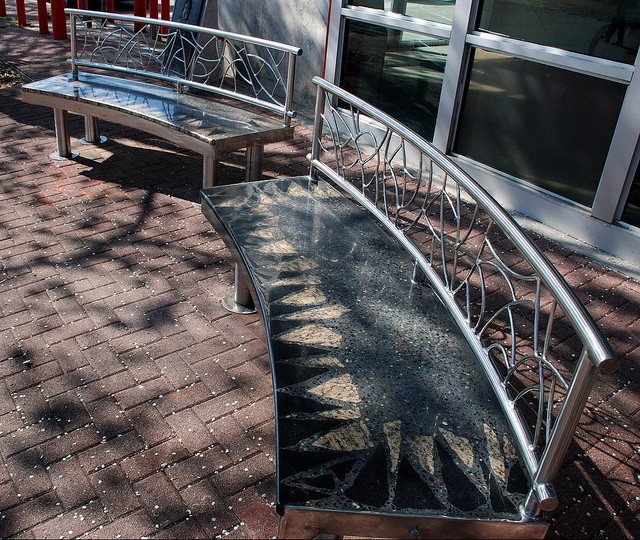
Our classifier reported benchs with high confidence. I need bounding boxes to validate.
[200,74,619,540]
[22,8,302,188]
